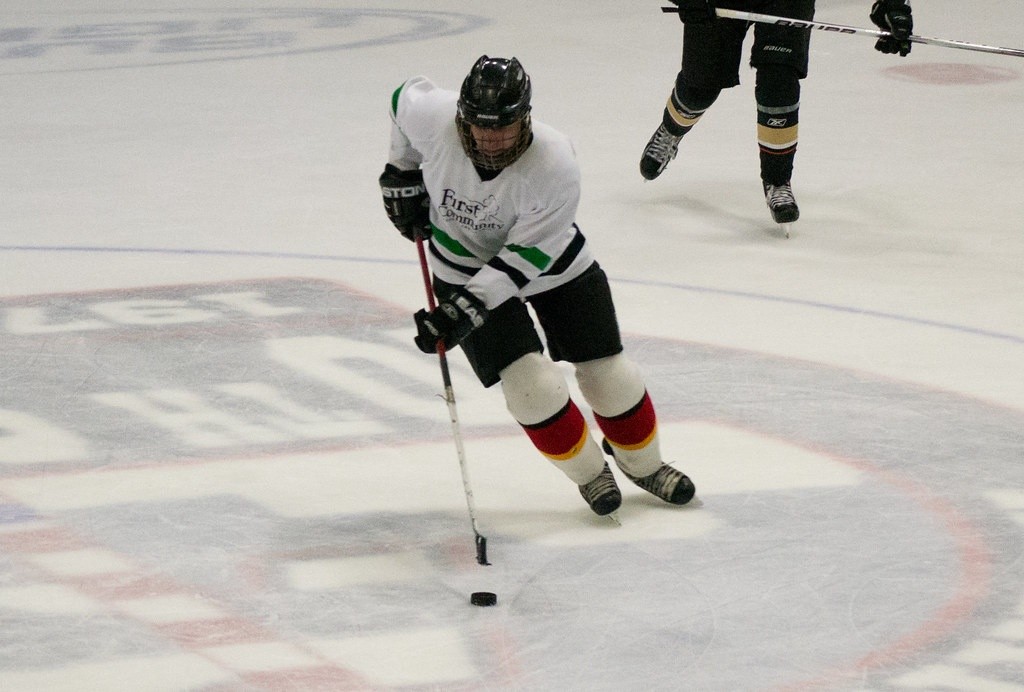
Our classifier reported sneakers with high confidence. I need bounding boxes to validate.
[639,121,683,183]
[602,438,696,506]
[578,461,622,527]
[763,180,800,238]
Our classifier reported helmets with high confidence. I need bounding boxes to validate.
[457,55,532,130]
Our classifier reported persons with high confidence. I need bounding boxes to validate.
[640,0,913,224]
[377,53,697,516]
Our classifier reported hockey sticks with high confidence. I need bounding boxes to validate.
[714,7,1024,60]
[411,227,500,565]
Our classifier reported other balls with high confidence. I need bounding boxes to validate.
[471,592,497,606]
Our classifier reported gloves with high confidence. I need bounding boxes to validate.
[668,0,717,26]
[379,172,433,242]
[870,0,913,57]
[413,287,489,354]
